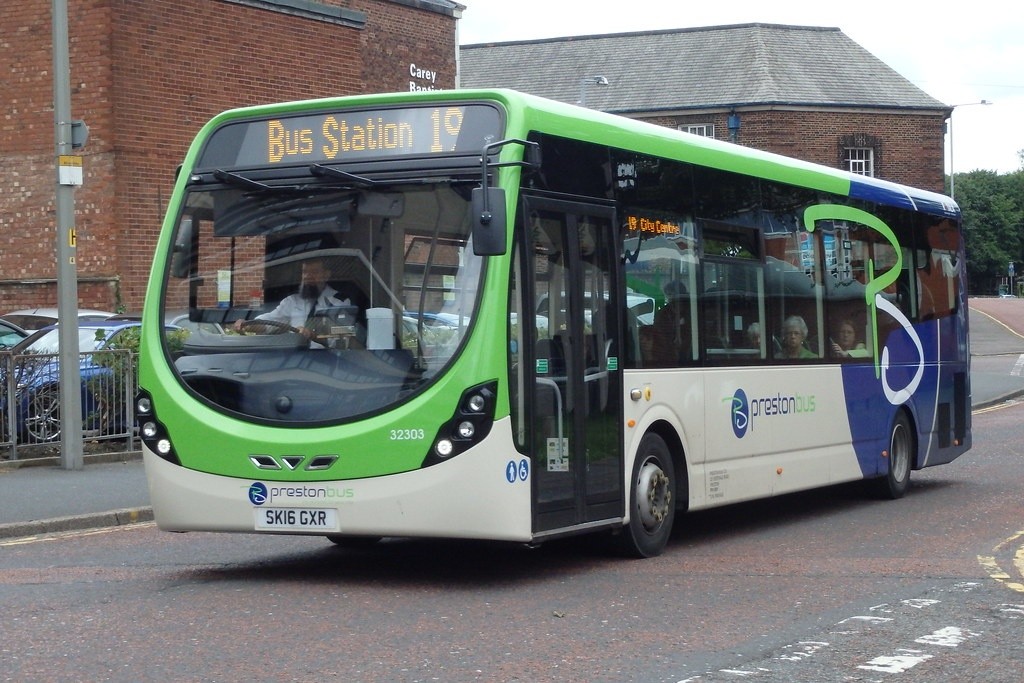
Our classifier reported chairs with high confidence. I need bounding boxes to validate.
[333,278,372,319]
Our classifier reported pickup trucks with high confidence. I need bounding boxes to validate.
[401,316,458,360]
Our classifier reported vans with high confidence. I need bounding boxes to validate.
[537,289,655,327]
[133,84,970,562]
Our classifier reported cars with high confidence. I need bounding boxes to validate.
[401,311,472,326]
[0,321,183,444]
[104,311,225,339]
[0,308,122,347]
[0,318,41,368]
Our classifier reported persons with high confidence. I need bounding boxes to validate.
[638,266,901,364]
[229,246,351,350]
